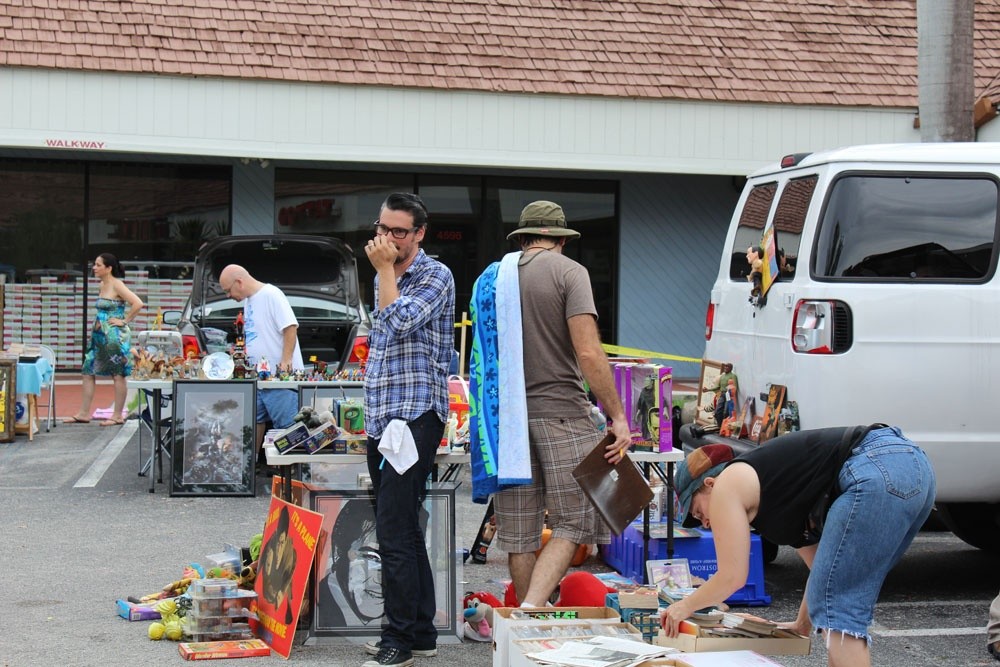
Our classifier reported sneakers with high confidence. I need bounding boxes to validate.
[364,642,416,666]
[365,636,436,656]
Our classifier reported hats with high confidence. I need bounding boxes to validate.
[671,446,734,528]
[507,201,581,243]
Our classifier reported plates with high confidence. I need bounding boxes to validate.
[204,351,235,380]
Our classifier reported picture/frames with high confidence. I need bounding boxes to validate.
[0,358,15,443]
[301,480,464,646]
[694,357,727,427]
[167,378,257,498]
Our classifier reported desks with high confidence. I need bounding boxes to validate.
[125,373,364,494]
[262,427,686,592]
[13,358,48,439]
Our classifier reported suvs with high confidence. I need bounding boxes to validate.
[161,233,372,381]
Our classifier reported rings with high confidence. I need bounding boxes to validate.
[368,244,374,247]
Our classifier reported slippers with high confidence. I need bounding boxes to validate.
[99,419,122,427]
[64,415,89,423]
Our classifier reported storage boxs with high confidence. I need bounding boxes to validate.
[176,541,272,661]
[303,361,339,372]
[598,352,672,454]
[490,512,813,667]
[200,327,231,353]
[258,394,369,455]
[0,268,194,370]
[115,598,161,621]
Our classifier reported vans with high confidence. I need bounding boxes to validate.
[698,140,1000,549]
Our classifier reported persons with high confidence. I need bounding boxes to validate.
[63,253,143,426]
[320,499,429,628]
[218,264,305,477]
[658,424,936,667]
[469,201,632,607]
[265,505,291,610]
[364,194,454,664]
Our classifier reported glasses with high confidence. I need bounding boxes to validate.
[223,281,237,294]
[374,221,419,239]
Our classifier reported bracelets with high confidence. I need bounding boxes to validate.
[122,319,127,325]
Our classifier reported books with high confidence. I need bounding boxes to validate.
[662,609,776,638]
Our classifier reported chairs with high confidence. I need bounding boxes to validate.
[133,388,171,477]
[25,343,57,432]
